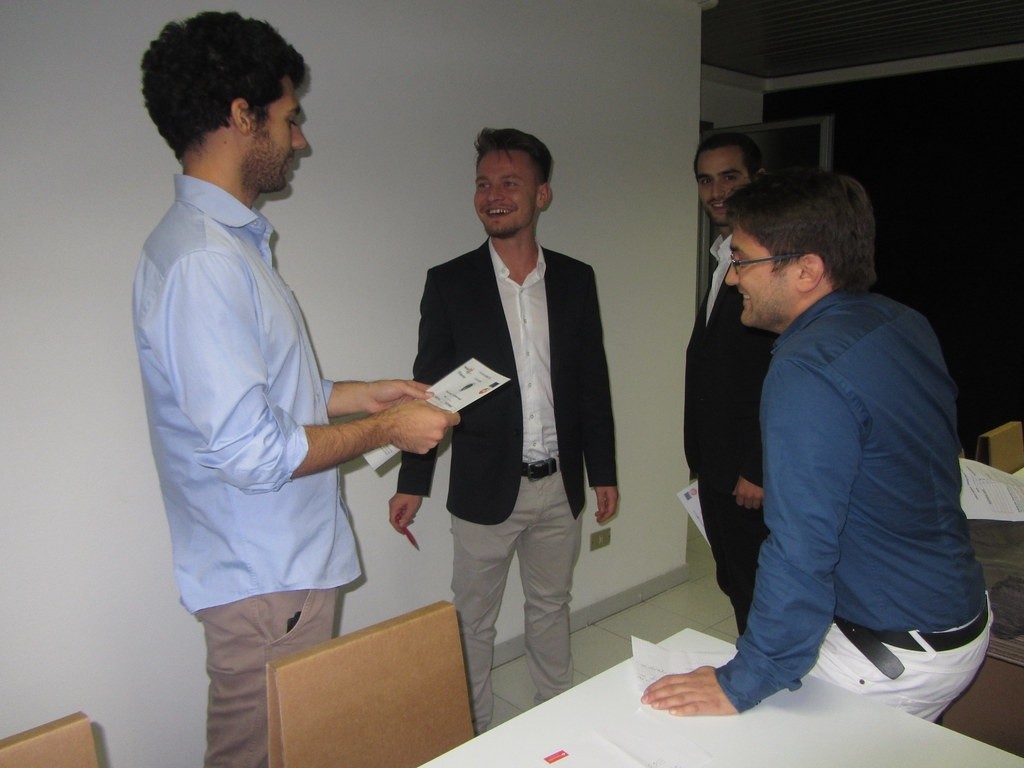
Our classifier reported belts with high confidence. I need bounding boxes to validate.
[835,592,997,686]
[520,456,559,481]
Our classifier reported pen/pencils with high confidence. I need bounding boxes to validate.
[395,515,422,552]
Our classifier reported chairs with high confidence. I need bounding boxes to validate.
[272,602,474,768]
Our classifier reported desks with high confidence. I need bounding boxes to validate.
[416,628,1024,768]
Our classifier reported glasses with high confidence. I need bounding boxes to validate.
[729,250,806,275]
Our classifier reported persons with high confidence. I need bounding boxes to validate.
[682,128,783,648]
[390,126,622,743]
[129,11,460,768]
[639,168,996,728]
